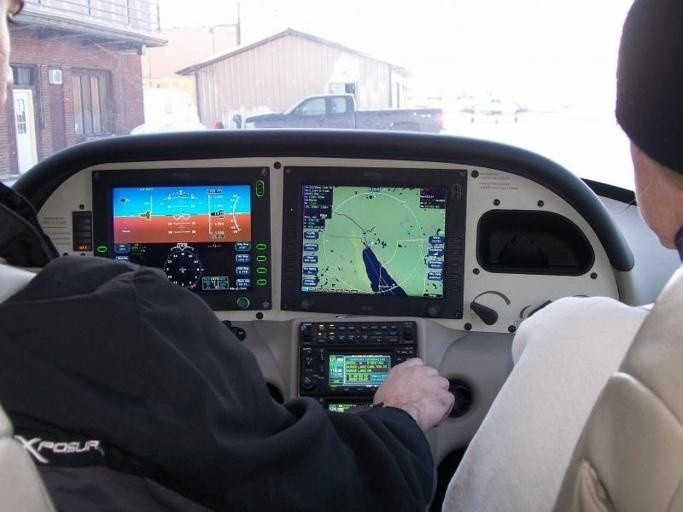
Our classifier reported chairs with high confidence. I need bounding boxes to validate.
[551,263,683,512]
[0,404,58,511]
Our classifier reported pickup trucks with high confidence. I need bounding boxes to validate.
[241,92,446,135]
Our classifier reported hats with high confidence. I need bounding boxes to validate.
[616,0,683,175]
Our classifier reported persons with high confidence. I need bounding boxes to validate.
[1,0,460,512]
[436,0,680,511]
